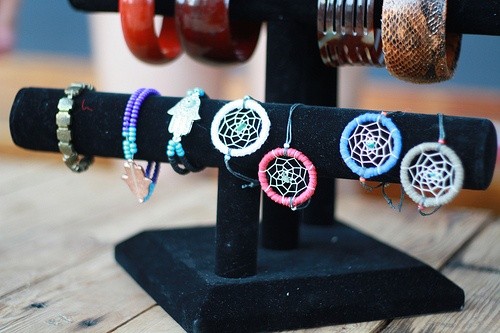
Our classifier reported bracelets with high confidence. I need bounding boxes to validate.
[175,0,264,67]
[401,112,466,217]
[167,84,211,175]
[339,110,402,212]
[382,0,464,84]
[317,0,386,69]
[260,103,318,211]
[123,87,167,206]
[120,0,186,67]
[56,83,96,173]
[212,95,272,188]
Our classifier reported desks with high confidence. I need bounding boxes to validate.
[0,154,500,333]
